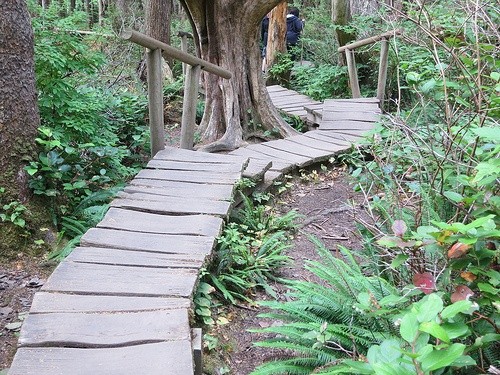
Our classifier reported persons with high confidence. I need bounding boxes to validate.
[261,12,268,72]
[286,7,305,68]
